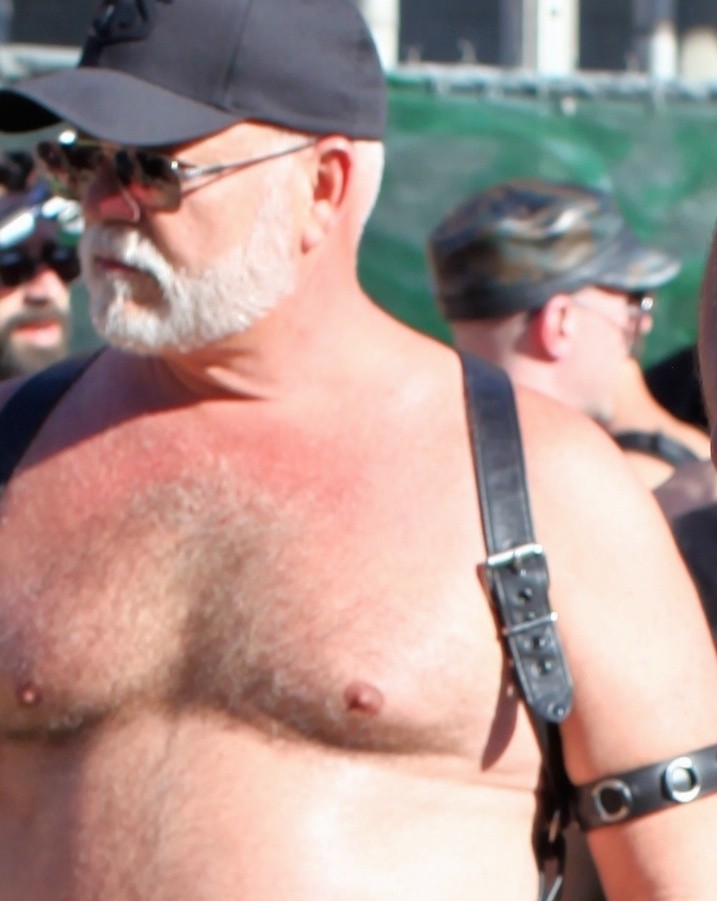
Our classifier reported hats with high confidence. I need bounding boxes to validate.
[0,0,388,148]
[426,181,683,322]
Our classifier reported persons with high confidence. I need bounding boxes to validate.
[0,0,717,901]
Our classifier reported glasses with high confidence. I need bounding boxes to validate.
[37,138,328,213]
[568,292,654,321]
[0,242,80,286]
